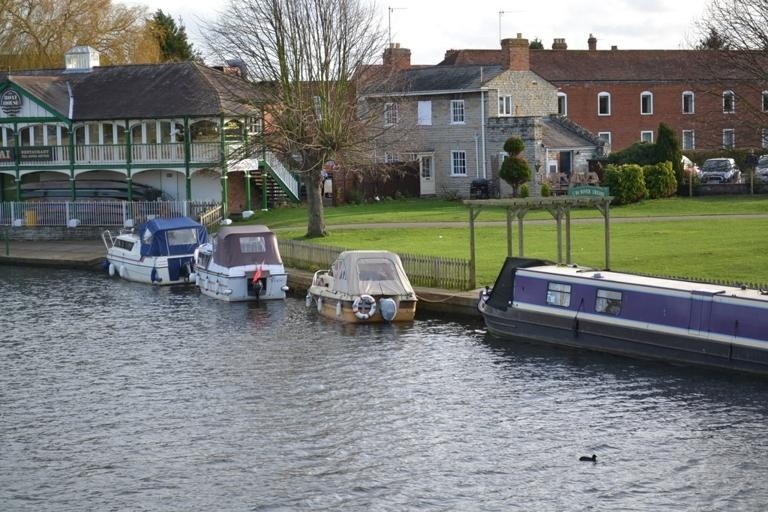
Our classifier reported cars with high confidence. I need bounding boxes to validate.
[697,157,740,184]
[680,155,699,180]
[752,155,768,192]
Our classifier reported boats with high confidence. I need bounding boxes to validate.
[104,215,209,286]
[478,256,767,381]
[193,222,289,303]
[307,249,417,324]
